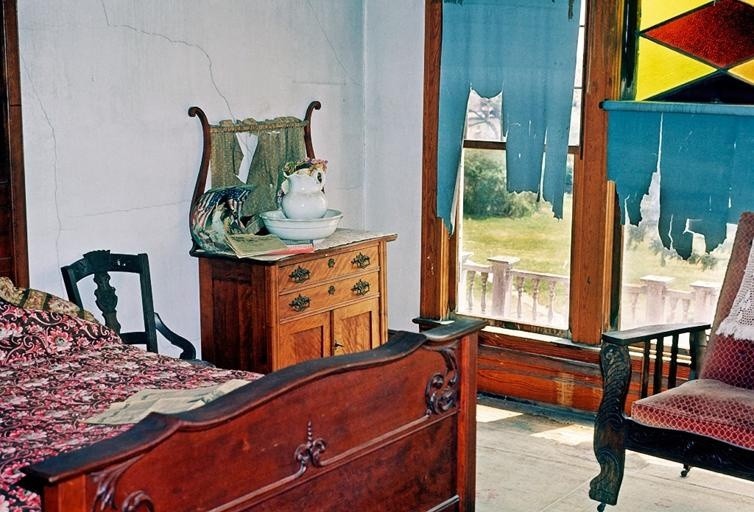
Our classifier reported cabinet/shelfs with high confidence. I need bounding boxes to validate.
[198,233,403,376]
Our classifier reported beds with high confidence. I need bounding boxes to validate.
[0,271,492,512]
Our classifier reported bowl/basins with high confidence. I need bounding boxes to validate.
[258,208,344,241]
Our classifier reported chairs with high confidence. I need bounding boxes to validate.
[59,249,197,365]
[586,210,752,511]
